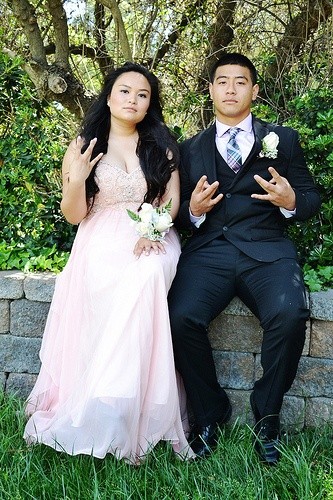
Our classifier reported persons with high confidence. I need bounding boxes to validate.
[166,53,322,465]
[23,60,197,466]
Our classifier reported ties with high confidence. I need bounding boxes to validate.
[225,126,245,174]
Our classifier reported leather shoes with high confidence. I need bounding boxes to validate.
[250,393,283,467]
[187,406,232,461]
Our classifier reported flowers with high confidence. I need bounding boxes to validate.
[259,132,279,160]
